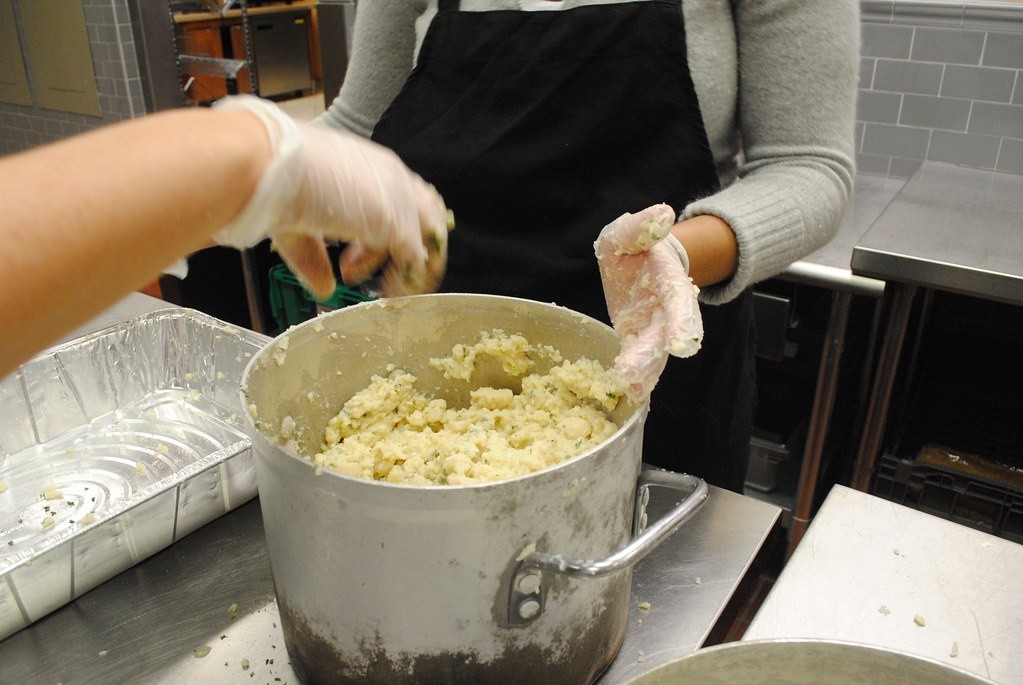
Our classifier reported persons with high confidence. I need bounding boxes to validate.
[297,3,863,499]
[1,93,454,384]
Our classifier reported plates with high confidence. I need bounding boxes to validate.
[1,307,279,645]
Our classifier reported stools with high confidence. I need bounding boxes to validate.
[728,485,1022,685]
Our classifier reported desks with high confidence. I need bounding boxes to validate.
[770,168,906,572]
[2,290,787,685]
[849,155,1022,543]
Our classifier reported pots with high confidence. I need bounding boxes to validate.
[238,293,711,685]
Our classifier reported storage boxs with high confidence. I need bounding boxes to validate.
[0,305,279,643]
